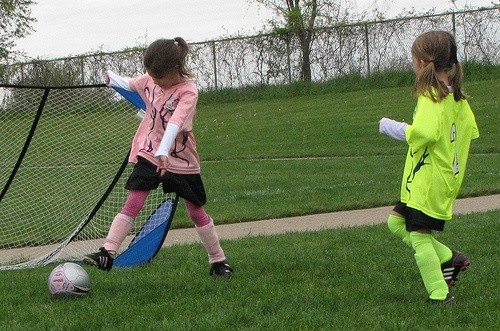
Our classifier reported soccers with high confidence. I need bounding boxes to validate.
[48,262,91,300]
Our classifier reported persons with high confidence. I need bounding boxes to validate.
[378,31,480,305]
[82,36,236,281]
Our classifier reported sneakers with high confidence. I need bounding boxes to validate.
[425,292,457,306]
[441,249,470,290]
[212,258,232,282]
[83,246,114,272]
